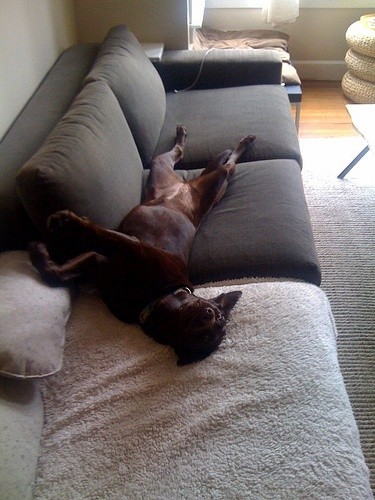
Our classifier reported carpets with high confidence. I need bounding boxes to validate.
[297,137,375,497]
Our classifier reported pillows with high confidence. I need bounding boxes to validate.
[14,79,146,266]
[82,24,166,170]
[0,249,73,381]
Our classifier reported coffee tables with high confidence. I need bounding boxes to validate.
[338,104,375,179]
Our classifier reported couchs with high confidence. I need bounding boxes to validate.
[0,40,375,500]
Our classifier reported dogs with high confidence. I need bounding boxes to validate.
[27,124,255,366]
